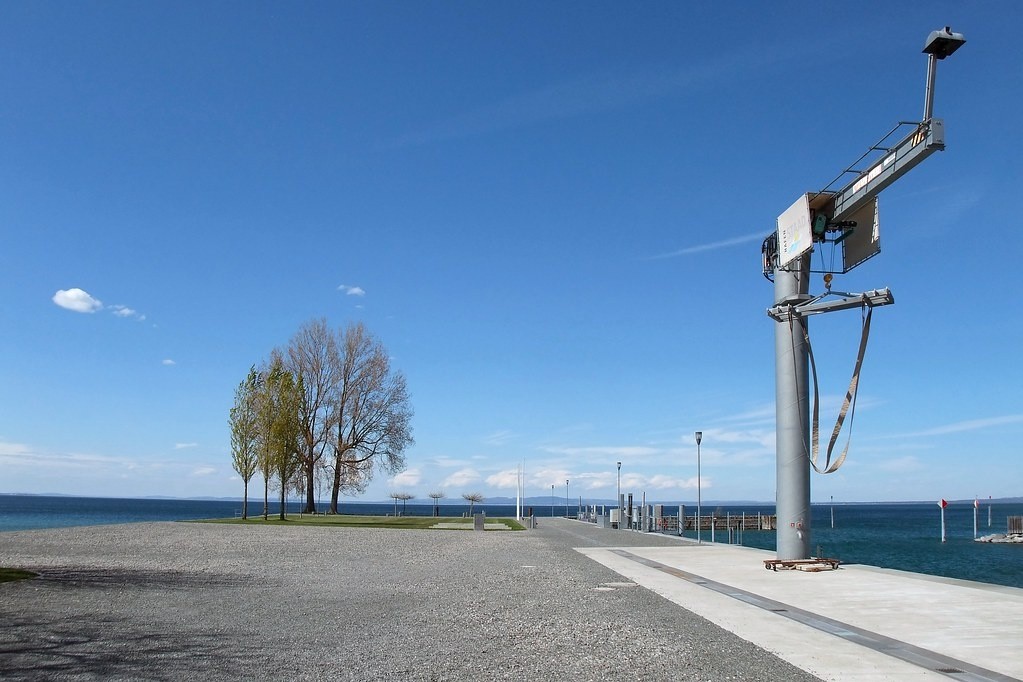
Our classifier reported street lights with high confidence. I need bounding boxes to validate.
[695,432,702,545]
[761,24,967,564]
[551,485,554,517]
[617,461,621,530]
[566,479,569,521]
